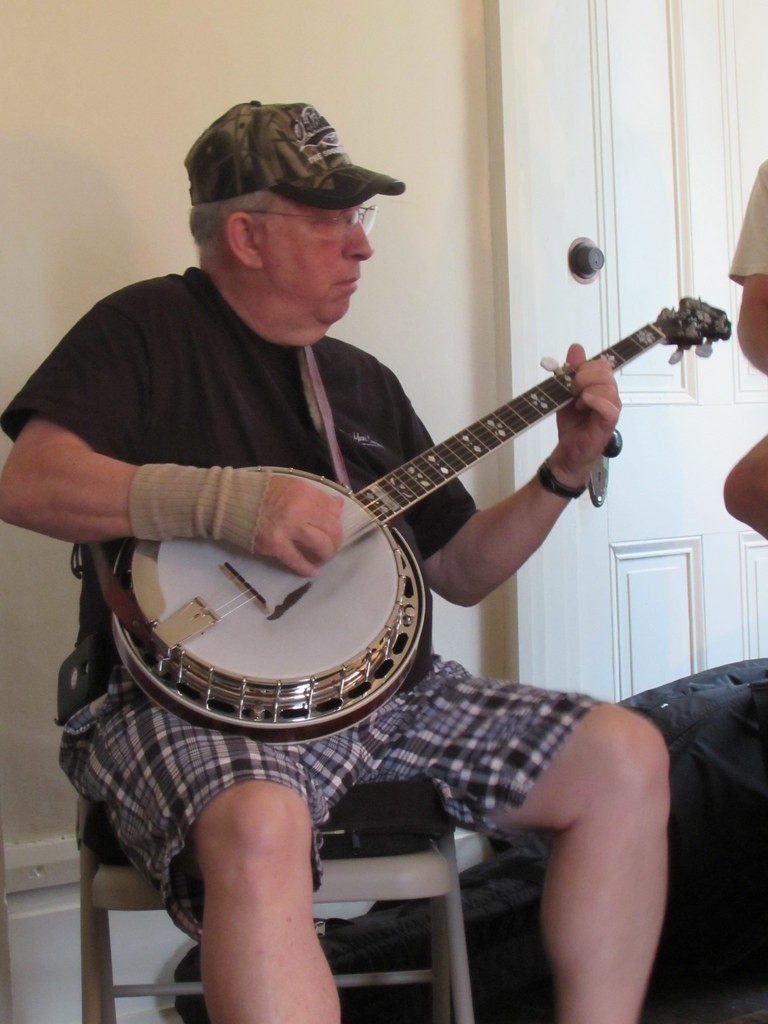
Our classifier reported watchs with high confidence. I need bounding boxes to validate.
[538,460,590,500]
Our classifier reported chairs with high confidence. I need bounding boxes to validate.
[73,790,476,1024]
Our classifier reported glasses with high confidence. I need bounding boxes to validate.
[245,204,378,236]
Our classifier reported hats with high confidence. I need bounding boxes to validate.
[183,101,406,208]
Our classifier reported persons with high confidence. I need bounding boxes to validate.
[723,156,768,541]
[0,102,670,1024]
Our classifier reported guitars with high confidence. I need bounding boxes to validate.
[110,294,730,742]
